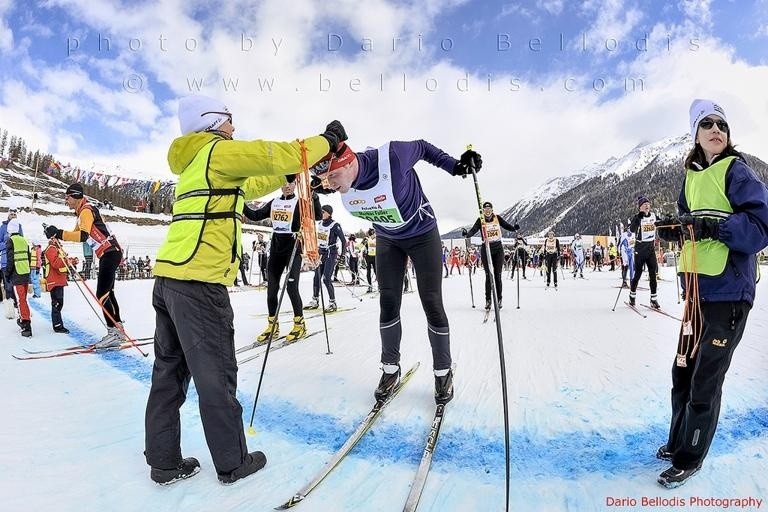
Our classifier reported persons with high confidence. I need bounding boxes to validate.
[629,196,663,309]
[309,139,482,403]
[245,177,323,341]
[305,204,345,312]
[49,184,125,349]
[655,100,767,489]
[144,95,347,485]
[1,237,150,300]
[461,202,520,309]
[1,211,24,304]
[2,219,33,338]
[234,226,634,296]
[42,225,78,334]
[263,185,267,188]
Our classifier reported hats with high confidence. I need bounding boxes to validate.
[321,204,333,215]
[178,91,231,137]
[483,201,493,208]
[636,196,650,206]
[66,181,82,199]
[691,98,731,147]
[6,208,19,234]
[308,138,356,176]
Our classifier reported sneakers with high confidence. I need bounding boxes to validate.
[304,298,338,313]
[375,362,400,402]
[152,457,200,486]
[347,278,372,292]
[17,319,69,337]
[547,282,558,287]
[32,295,41,299]
[258,317,279,342]
[650,293,660,309]
[215,451,268,486]
[434,369,454,405]
[286,316,306,341]
[485,299,503,310]
[656,441,702,488]
[629,293,637,305]
[93,322,124,350]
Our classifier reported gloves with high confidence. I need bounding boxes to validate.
[455,148,482,176]
[320,119,348,153]
[653,215,721,243]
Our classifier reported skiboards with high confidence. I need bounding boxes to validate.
[625,301,682,324]
[483,308,489,323]
[235,329,327,364]
[251,304,357,323]
[13,336,155,360]
[276,363,457,511]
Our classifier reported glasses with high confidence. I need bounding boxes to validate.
[64,193,79,199]
[305,147,348,174]
[200,111,233,123]
[698,116,729,133]
